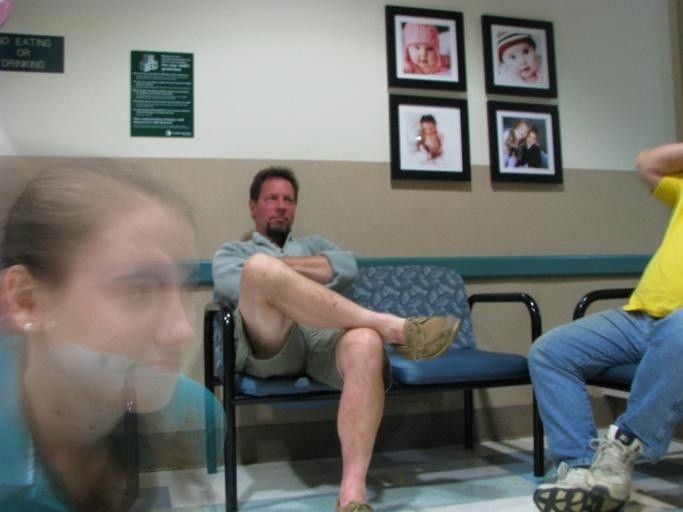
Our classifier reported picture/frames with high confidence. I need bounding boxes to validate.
[486,99,564,184]
[481,12,558,98]
[384,3,467,91]
[389,93,471,182]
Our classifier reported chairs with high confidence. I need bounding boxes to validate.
[203,265,548,512]
[569,287,683,394]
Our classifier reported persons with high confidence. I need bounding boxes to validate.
[416,113,449,163]
[0,158,227,512]
[496,28,546,84]
[212,166,460,512]
[528,140,683,511]
[402,23,450,75]
[503,120,550,168]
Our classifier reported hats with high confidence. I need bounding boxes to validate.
[403,23,442,72]
[496,31,536,64]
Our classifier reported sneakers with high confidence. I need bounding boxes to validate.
[334,496,374,512]
[585,423,646,502]
[391,315,461,361]
[532,461,631,511]
